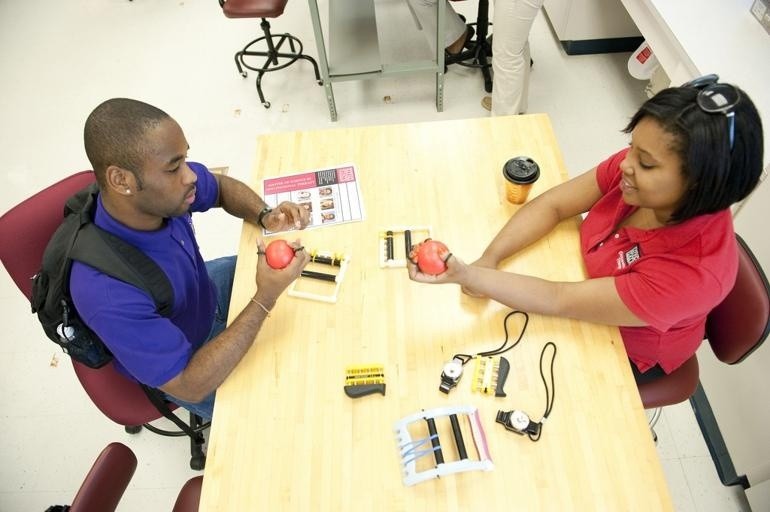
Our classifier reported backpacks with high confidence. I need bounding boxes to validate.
[30,185,175,369]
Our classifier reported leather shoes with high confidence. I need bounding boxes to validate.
[482,96,492,107]
[444,25,474,63]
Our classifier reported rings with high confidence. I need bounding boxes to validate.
[256,251,266,255]
[293,246,305,252]
[444,252,452,265]
[408,244,419,264]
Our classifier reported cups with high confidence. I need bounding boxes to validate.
[503,155,541,205]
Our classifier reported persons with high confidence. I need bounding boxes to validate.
[403,1,544,118]
[70,96,311,425]
[296,187,337,227]
[405,72,764,383]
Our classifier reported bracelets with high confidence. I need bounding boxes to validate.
[251,298,270,314]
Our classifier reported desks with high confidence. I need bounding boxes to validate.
[306,1,454,126]
[167,111,680,512]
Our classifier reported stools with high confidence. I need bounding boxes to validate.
[442,0,535,97]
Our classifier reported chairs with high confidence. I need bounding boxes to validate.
[626,229,770,449]
[0,168,208,472]
[216,0,325,114]
[64,440,204,512]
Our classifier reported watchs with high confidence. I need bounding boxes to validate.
[258,206,272,229]
[497,407,542,438]
[440,355,465,393]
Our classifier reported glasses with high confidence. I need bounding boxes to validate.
[687,73,740,152]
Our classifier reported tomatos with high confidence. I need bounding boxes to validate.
[265,239,294,270]
[417,240,451,276]
